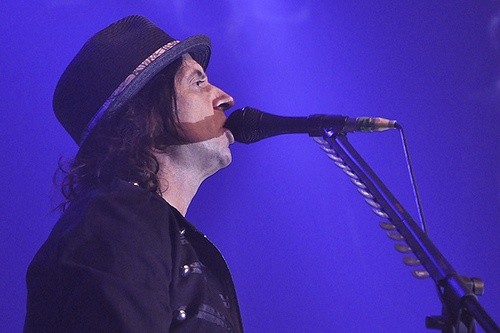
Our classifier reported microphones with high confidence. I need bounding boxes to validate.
[228,106,398,144]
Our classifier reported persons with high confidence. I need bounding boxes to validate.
[23,16,245,333]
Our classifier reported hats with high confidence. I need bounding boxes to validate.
[52,14,212,155]
